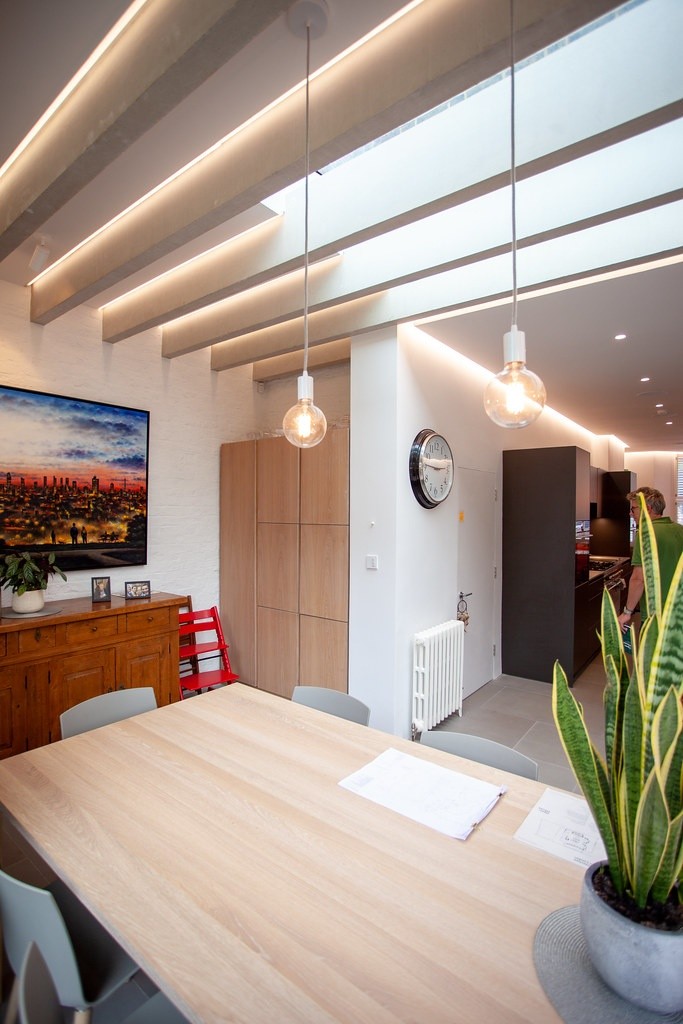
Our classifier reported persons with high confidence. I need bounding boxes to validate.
[95,582,109,601]
[127,584,149,597]
[51,523,116,544]
[617,487,683,634]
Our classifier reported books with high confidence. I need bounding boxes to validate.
[621,624,633,655]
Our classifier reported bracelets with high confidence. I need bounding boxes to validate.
[623,606,633,615]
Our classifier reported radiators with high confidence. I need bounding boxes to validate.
[413,618,462,742]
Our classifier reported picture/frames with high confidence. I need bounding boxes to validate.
[92,577,112,603]
[124,581,151,600]
[0,384,151,570]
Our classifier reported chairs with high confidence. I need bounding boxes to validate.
[291,686,370,727]
[1,872,141,1024]
[177,607,240,700]
[420,731,539,783]
[59,687,160,741]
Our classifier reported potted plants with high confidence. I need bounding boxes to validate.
[1,552,67,613]
[551,490,683,1013]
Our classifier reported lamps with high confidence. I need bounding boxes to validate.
[484,0,549,427]
[282,1,327,448]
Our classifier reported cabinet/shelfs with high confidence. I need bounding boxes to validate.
[0,589,194,758]
[502,446,636,689]
[219,424,349,701]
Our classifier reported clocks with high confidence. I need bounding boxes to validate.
[409,428,454,509]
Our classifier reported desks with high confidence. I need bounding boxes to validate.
[0,682,596,1023]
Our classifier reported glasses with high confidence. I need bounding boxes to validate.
[630,506,639,513]
